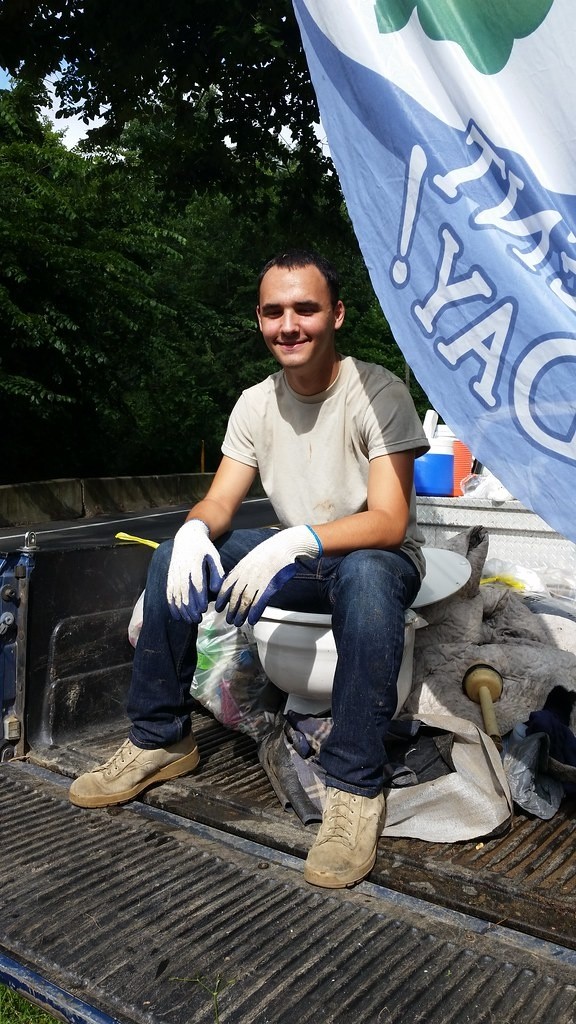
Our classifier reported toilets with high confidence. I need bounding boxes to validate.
[252,606,416,726]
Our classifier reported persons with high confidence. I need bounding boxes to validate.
[69,254,432,888]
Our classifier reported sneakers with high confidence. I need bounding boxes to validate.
[303,786,387,888]
[69,730,199,808]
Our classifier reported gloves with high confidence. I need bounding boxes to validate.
[166,518,226,625]
[214,523,323,627]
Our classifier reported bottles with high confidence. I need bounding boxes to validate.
[191,626,220,696]
